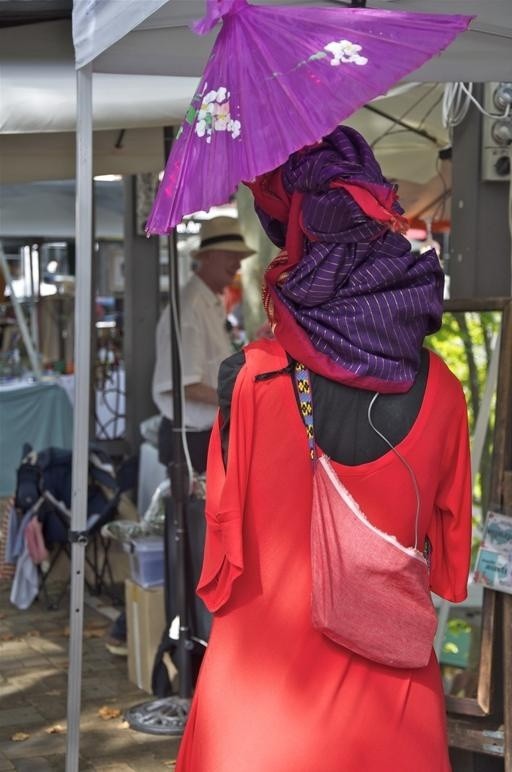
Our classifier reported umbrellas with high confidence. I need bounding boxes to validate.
[143,1,474,241]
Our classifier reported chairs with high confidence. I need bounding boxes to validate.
[8,451,137,612]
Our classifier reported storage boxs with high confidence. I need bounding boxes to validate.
[123,577,168,691]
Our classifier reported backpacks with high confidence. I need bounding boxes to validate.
[15,443,119,549]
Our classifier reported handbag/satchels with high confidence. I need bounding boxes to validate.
[312,456,438,669]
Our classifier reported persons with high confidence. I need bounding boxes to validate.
[173,125,475,771]
[149,214,257,700]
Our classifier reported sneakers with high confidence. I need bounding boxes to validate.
[106,638,128,655]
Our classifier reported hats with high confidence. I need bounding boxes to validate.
[190,217,257,259]
